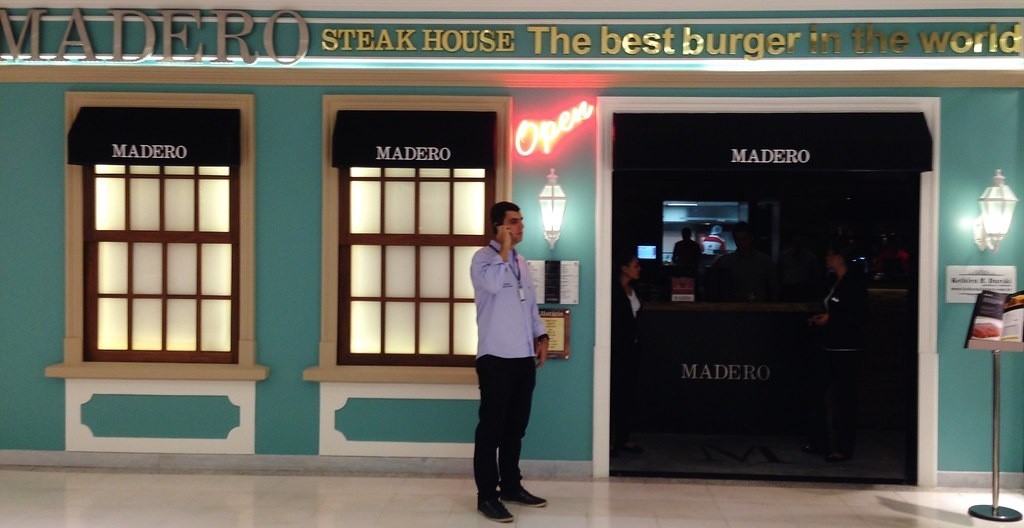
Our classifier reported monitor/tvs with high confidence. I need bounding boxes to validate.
[637,245,657,260]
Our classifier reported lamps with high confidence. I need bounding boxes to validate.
[973,169,1020,255]
[538,168,568,251]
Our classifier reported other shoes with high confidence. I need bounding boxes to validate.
[825,440,853,463]
[801,438,828,453]
[611,443,618,458]
[615,437,637,450]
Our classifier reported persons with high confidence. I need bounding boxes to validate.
[470,201,549,522]
[672,227,705,266]
[802,235,868,463]
[610,250,642,459]
[705,221,774,303]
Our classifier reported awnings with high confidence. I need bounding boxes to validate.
[67,106,241,167]
[613,112,932,173]
[332,110,497,168]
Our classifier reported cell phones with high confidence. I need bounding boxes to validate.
[493,224,501,234]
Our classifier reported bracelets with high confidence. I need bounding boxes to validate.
[537,334,549,344]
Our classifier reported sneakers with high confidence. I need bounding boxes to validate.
[500,487,547,507]
[476,496,514,522]
